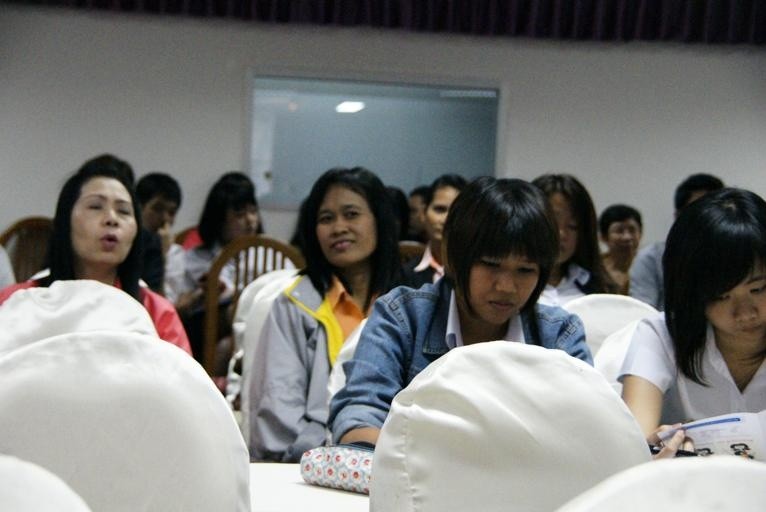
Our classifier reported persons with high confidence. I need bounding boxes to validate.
[249,167,412,464]
[617,186,766,436]
[410,174,723,310]
[0,155,270,375]
[328,176,594,442]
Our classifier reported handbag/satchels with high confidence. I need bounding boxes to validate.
[299,441,375,494]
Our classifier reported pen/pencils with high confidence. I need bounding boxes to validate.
[649,444,698,457]
